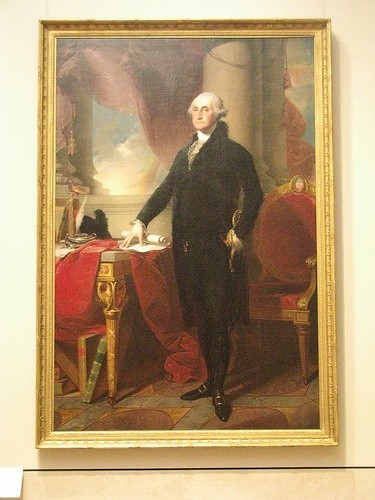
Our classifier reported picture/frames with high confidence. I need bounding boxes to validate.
[35,19,339,449]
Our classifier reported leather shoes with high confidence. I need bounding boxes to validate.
[180,381,209,400]
[209,393,228,424]
